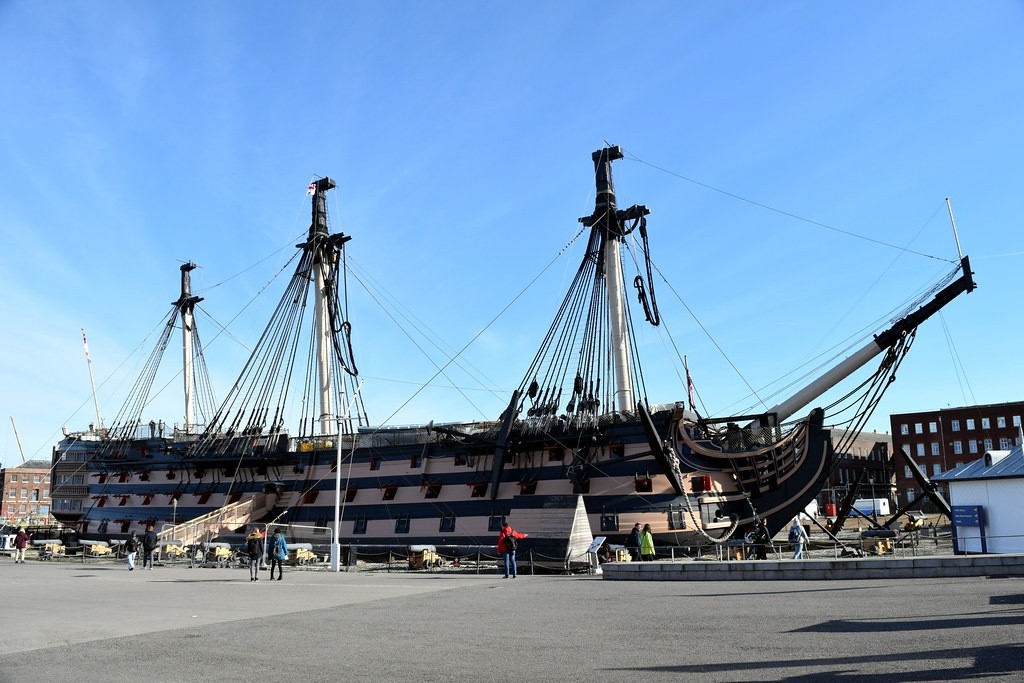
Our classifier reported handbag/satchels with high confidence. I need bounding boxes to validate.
[148,538,157,549]
[268,546,280,560]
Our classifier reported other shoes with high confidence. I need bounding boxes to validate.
[15,561,18,563]
[513,576,516,579]
[270,577,275,580]
[277,577,282,580]
[148,568,153,570]
[130,568,133,571]
[503,576,509,579]
[255,577,258,580]
[21,561,25,563]
[251,578,254,581]
[141,567,145,569]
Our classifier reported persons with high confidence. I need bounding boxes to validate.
[624,522,655,561]
[744,517,772,560]
[268,527,288,580]
[141,525,158,569]
[147,419,165,438]
[13,527,30,563]
[124,530,140,571]
[246,527,264,582]
[790,518,809,559]
[497,522,529,578]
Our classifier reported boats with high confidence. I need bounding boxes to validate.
[861,529,899,538]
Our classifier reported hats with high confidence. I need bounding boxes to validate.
[274,528,281,533]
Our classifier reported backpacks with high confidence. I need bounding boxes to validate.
[624,536,632,550]
[500,530,517,551]
[789,527,801,543]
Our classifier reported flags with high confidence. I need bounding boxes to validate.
[82,331,91,362]
[688,375,696,408]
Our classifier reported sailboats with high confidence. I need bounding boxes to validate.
[47,145,978,575]
